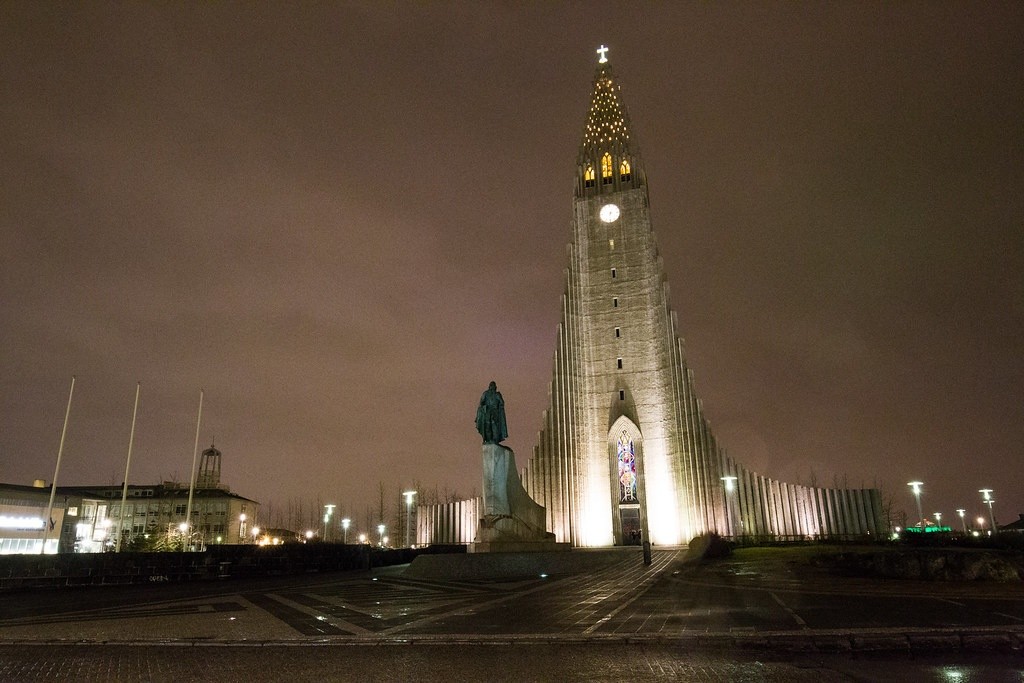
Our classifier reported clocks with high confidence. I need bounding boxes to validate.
[598,203,621,223]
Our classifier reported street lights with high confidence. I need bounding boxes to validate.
[955,509,968,537]
[402,491,418,548]
[239,513,246,542]
[342,519,351,545]
[977,517,984,535]
[907,481,926,533]
[978,488,998,534]
[933,512,943,533]
[322,504,336,543]
[720,475,739,545]
[378,524,385,543]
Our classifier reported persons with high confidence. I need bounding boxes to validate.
[474,380,508,446]
